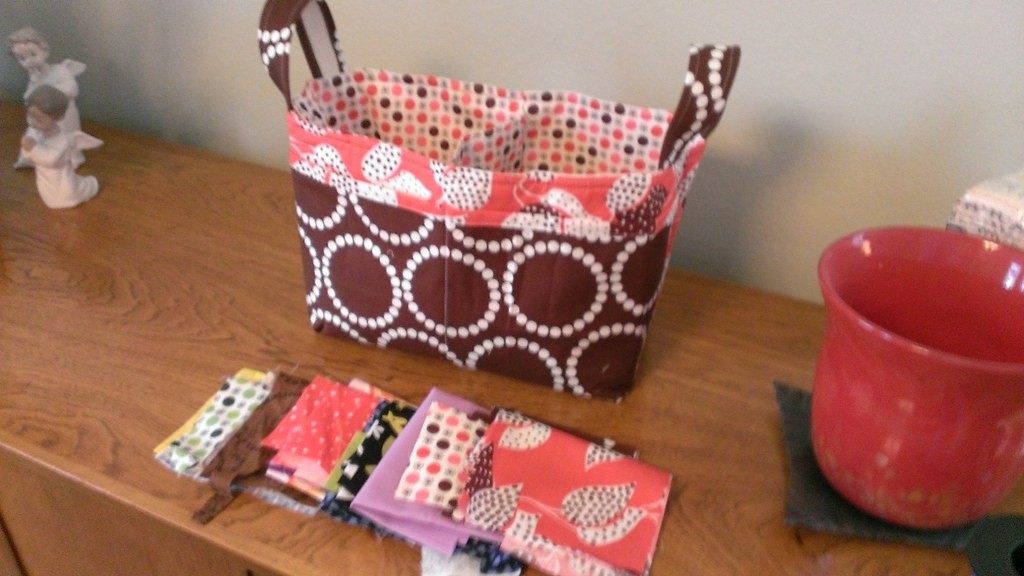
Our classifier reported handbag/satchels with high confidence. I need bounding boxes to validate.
[258,0,742,402]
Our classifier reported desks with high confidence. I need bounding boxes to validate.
[0,101,1024,576]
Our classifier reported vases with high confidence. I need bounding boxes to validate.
[810,225,1024,532]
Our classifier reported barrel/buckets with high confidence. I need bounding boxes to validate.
[812,226,1023,529]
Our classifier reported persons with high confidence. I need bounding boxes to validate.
[5,27,104,209]
